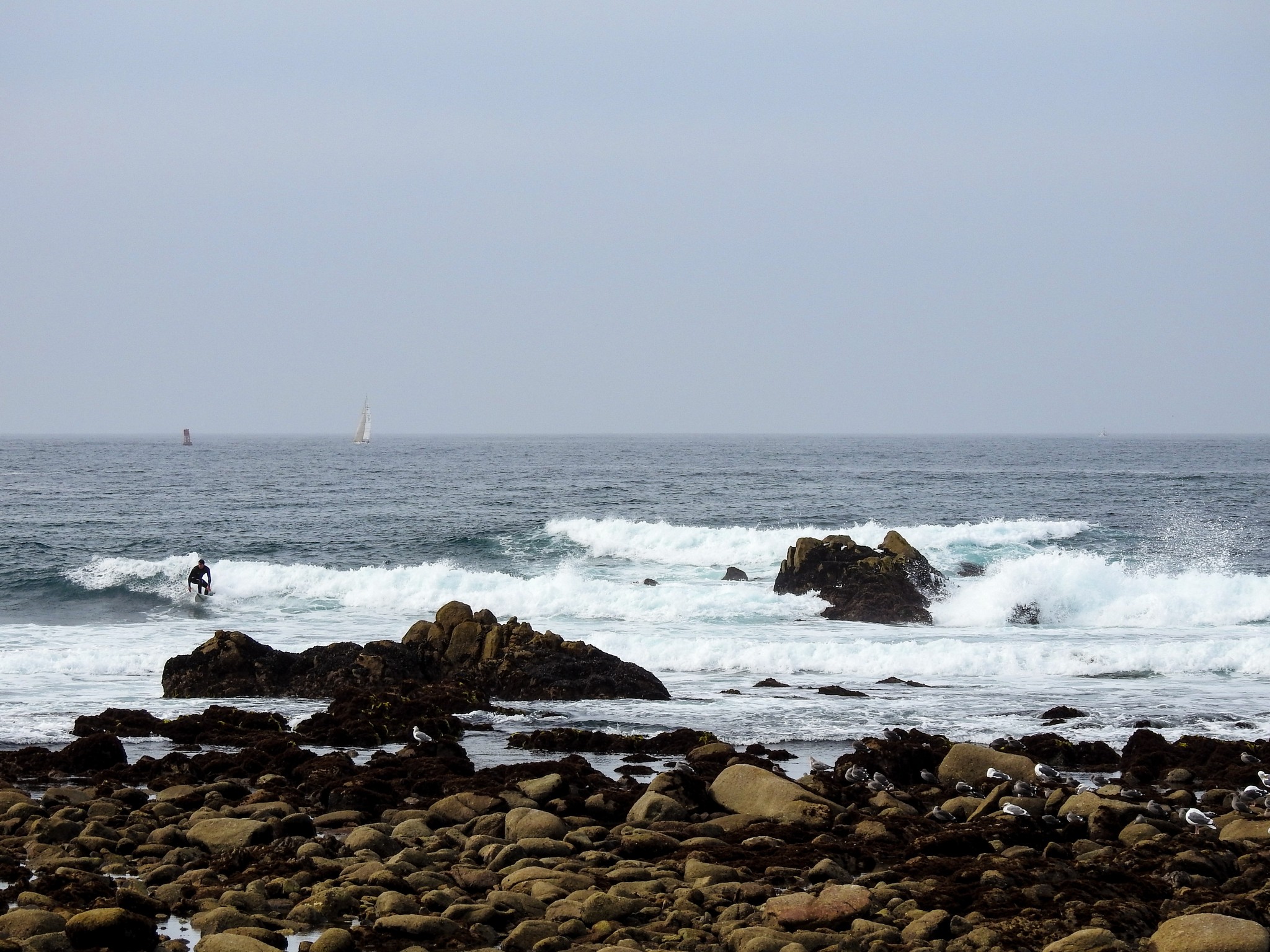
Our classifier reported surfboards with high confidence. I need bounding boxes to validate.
[195,593,209,603]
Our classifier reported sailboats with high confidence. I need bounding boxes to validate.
[352,394,372,444]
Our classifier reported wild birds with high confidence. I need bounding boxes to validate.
[1258,771,1270,788]
[1229,793,1259,815]
[1066,777,1081,787]
[413,726,438,746]
[920,769,943,785]
[865,779,887,791]
[845,767,857,783]
[1075,783,1105,799]
[1121,787,1145,801]
[1090,775,1113,790]
[808,757,835,775]
[1014,780,1037,797]
[1185,807,1222,835]
[986,768,1012,780]
[1178,808,1189,826]
[1035,763,1068,783]
[956,782,985,798]
[1134,814,1147,824]
[1001,802,1031,817]
[675,761,697,774]
[1147,800,1170,821]
[1065,810,1088,823]
[887,783,912,798]
[1236,785,1267,802]
[989,738,1008,750]
[1043,788,1055,798]
[881,728,901,740]
[1240,752,1261,764]
[1007,736,1028,752]
[852,739,872,753]
[874,772,889,786]
[852,764,870,780]
[933,806,957,822]
[1041,815,1061,824]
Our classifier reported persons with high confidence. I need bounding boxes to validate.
[188,559,211,596]
